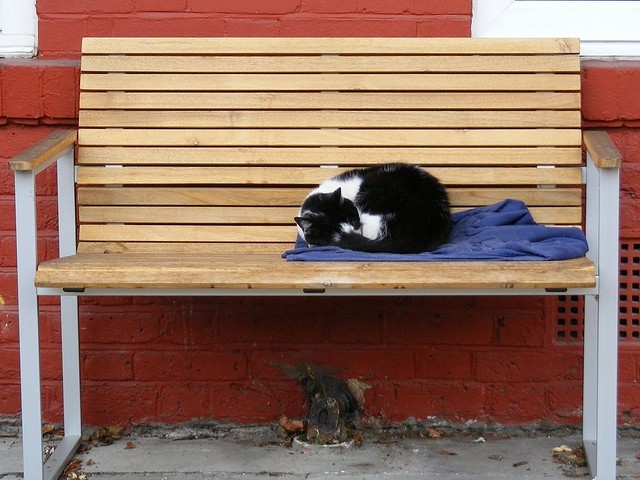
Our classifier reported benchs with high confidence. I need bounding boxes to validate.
[7,36,624,480]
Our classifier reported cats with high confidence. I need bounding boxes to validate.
[294,161,454,256]
